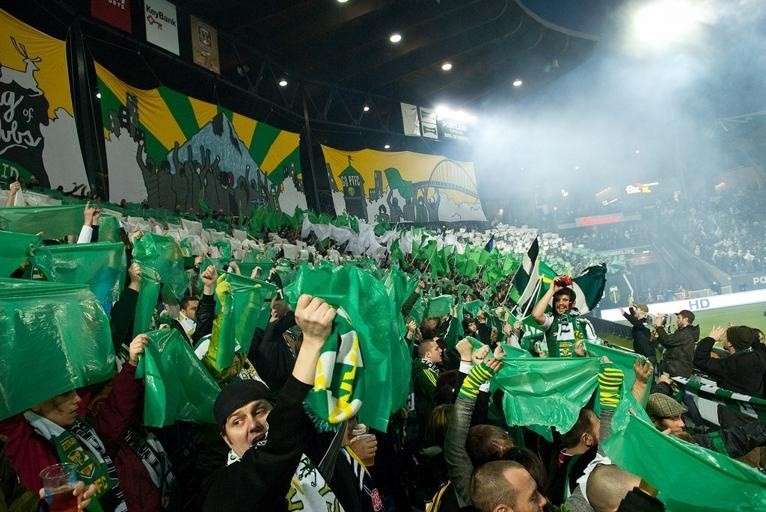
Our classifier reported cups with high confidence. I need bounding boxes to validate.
[39,461,79,511]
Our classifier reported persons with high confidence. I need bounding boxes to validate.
[0,175,766,512]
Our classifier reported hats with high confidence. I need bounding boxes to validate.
[727,326,754,349]
[633,303,649,313]
[212,379,275,434]
[646,392,688,418]
[675,310,695,320]
